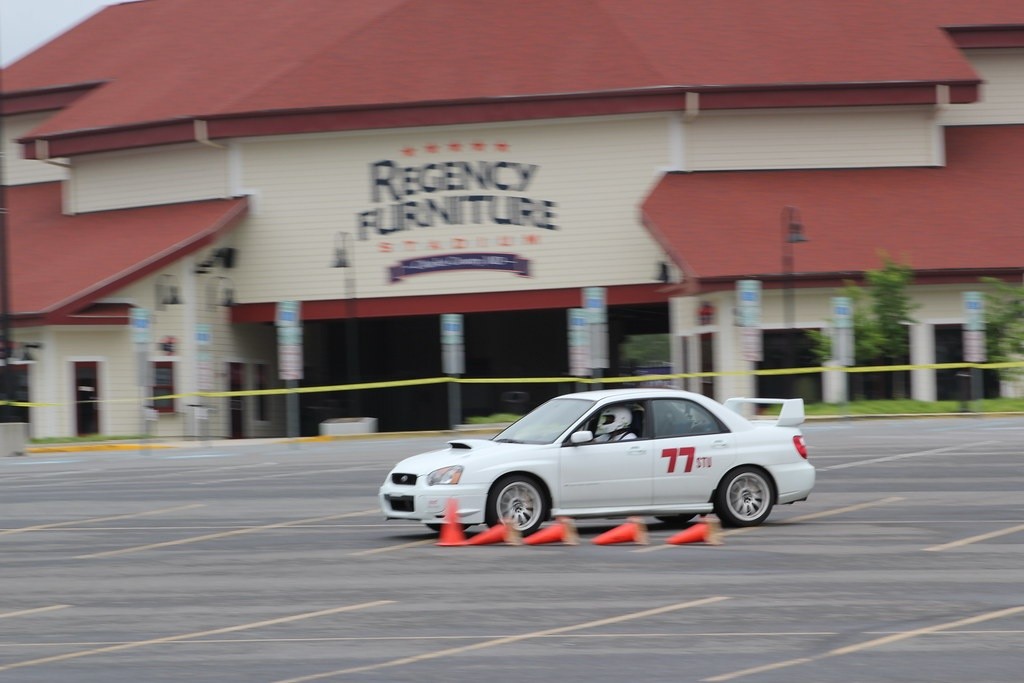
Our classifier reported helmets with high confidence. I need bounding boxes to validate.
[594,406,632,435]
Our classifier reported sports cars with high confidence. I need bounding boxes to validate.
[377,387,817,538]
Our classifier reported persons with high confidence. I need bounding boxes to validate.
[588,405,637,443]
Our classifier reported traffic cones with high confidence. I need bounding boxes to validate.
[665,514,724,546]
[463,517,518,546]
[433,497,466,547]
[520,516,580,547]
[589,515,649,545]
